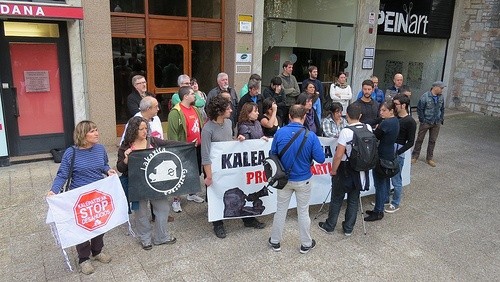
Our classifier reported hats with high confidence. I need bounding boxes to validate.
[431,81,447,87]
[270,77,283,86]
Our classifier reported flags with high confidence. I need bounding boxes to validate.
[128,143,201,202]
[44,174,130,250]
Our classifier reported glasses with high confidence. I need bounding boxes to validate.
[135,81,147,84]
[181,82,191,85]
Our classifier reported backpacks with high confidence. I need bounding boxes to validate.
[343,123,378,171]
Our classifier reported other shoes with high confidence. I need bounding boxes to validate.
[364,210,384,222]
[371,201,399,213]
[244,219,266,228]
[214,224,226,238]
[152,214,174,222]
[426,159,436,166]
[79,259,94,274]
[141,240,153,250]
[342,221,352,237]
[154,237,177,245]
[318,221,333,234]
[411,157,416,163]
[91,252,112,263]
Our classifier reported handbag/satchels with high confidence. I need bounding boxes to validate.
[376,159,399,178]
[262,156,288,190]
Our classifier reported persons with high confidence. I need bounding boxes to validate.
[200,96,266,238]
[167,86,204,213]
[117,61,417,254]
[330,72,352,116]
[46,120,117,274]
[411,81,448,167]
[236,102,264,140]
[269,104,325,254]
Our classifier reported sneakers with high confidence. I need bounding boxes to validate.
[172,197,183,212]
[268,236,281,252]
[300,239,316,253]
[187,192,204,203]
